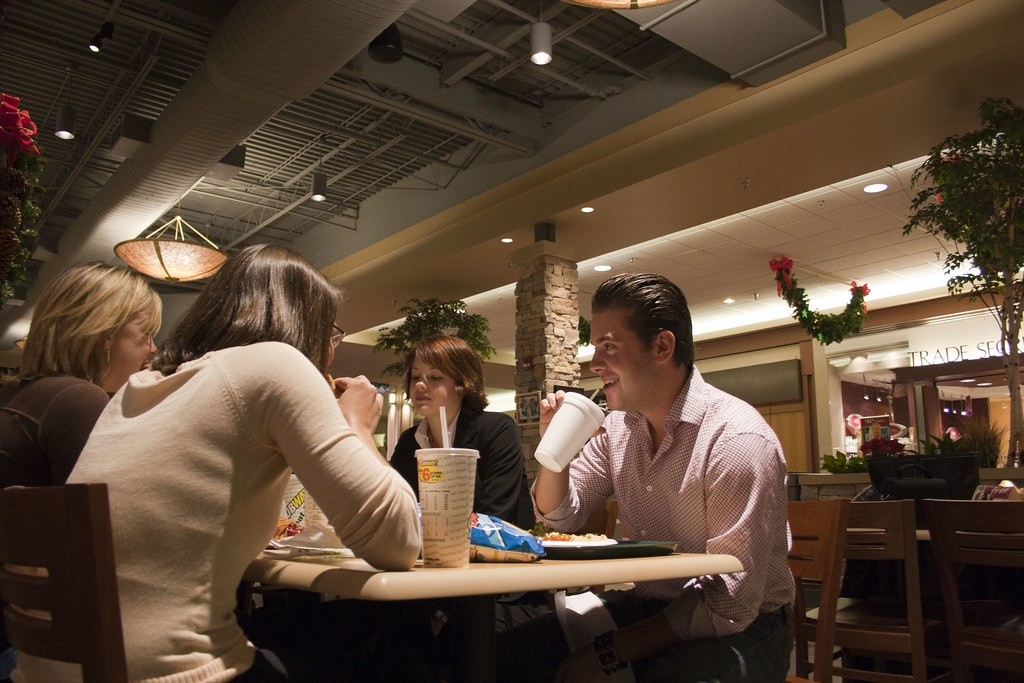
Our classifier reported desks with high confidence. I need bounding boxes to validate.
[240,547,745,683]
[841,526,1024,683]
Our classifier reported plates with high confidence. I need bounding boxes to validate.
[540,539,618,547]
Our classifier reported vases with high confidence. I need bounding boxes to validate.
[860,454,981,500]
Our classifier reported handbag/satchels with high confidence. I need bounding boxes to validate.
[890,463,952,560]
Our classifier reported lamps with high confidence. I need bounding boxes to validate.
[942,390,967,415]
[14,336,27,351]
[875,380,882,402]
[368,23,403,66]
[531,0,554,65]
[863,374,871,400]
[89,21,114,53]
[310,135,329,202]
[55,73,78,139]
[114,199,229,284]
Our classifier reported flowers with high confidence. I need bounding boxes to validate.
[859,434,962,456]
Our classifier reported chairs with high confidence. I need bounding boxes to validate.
[0,483,129,683]
[793,499,951,683]
[922,499,1024,683]
[785,497,853,683]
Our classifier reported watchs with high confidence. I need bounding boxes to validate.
[593,629,627,676]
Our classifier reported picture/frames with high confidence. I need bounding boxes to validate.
[514,390,542,426]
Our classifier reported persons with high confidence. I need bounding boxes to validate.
[493,273,799,683]
[387,334,535,535]
[0,259,164,616]
[62,244,422,683]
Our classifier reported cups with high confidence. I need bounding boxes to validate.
[415,447,480,569]
[284,473,330,531]
[533,392,605,472]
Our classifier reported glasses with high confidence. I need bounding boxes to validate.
[331,323,348,351]
